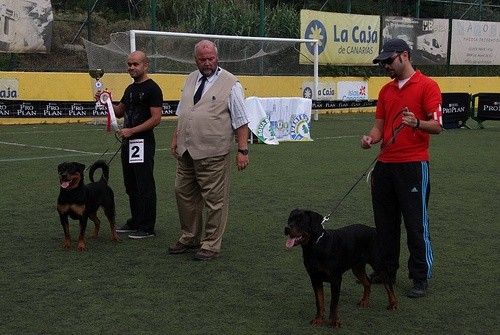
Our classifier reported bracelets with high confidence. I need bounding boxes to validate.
[413,117,419,129]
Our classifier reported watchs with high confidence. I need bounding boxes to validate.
[238,149,248,155]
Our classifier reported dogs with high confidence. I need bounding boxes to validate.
[57,160,123,251]
[283,208,400,328]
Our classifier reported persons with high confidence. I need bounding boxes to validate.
[168,40,249,260]
[360,40,443,298]
[94,51,164,238]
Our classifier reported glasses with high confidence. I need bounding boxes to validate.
[379,52,402,67]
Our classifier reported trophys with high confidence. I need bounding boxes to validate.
[88,69,105,108]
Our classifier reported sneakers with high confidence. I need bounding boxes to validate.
[116,223,137,233]
[128,229,155,239]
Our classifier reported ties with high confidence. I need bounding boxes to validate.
[194,76,207,105]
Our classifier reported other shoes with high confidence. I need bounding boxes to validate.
[355,271,396,286]
[168,241,200,255]
[406,284,428,298]
[193,248,219,260]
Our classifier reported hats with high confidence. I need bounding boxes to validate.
[373,38,410,65]
